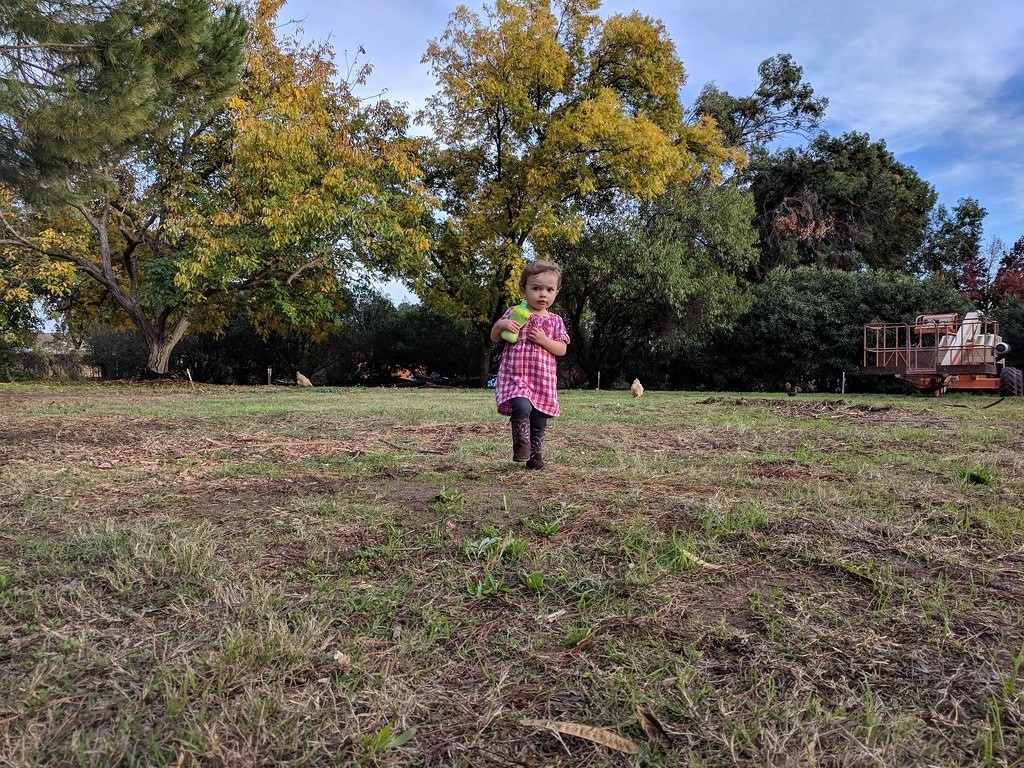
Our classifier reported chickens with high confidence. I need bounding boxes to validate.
[296,371,314,387]
[630,379,643,398]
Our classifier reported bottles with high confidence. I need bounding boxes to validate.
[501,301,530,343]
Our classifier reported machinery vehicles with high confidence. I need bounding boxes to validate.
[862,308,1023,398]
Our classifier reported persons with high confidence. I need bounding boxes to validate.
[490,259,570,469]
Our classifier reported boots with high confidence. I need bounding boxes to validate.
[526,432,545,470]
[510,416,530,462]
